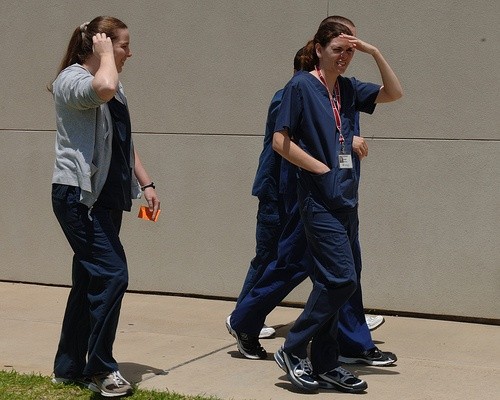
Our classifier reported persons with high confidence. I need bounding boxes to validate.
[272,23,403,396]
[236,47,384,339]
[339,156,344,163]
[225,16,398,366]
[47,16,160,397]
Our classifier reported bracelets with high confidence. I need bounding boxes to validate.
[141,182,156,191]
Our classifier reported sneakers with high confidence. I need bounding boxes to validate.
[225,313,267,360]
[310,359,368,394]
[228,309,276,339]
[89,370,133,397]
[274,343,320,392]
[365,314,386,331]
[338,344,397,366]
[51,369,93,387]
